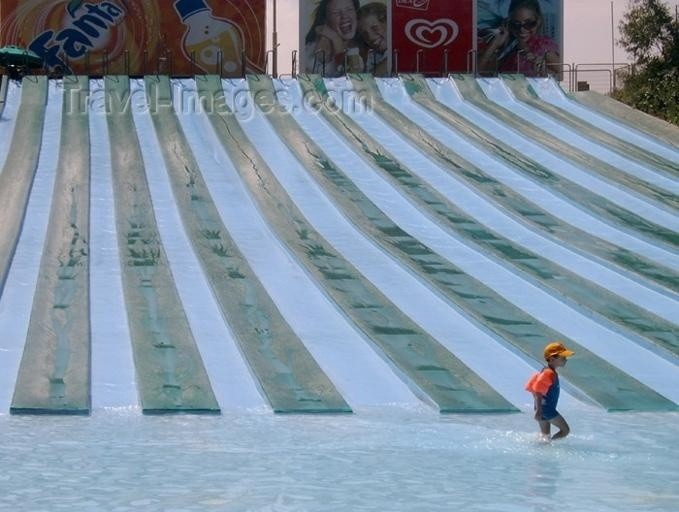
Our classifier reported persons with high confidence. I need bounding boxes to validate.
[304,0,368,80]
[525,339,576,448]
[356,2,389,77]
[478,0,561,80]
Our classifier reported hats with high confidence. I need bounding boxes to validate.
[543,341,576,362]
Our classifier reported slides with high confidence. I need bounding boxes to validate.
[3,74,679,416]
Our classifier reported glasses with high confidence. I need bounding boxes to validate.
[506,16,538,34]
